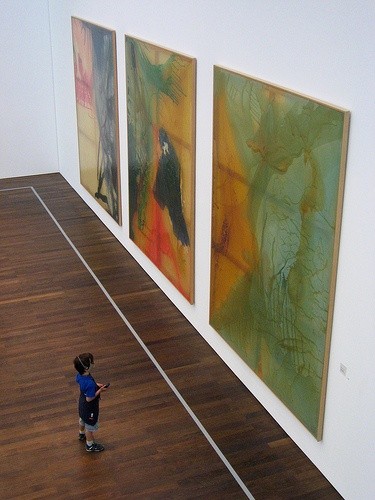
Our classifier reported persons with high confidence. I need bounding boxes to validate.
[73,352,108,451]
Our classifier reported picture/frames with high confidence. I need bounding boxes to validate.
[70,16,121,229]
[116,34,200,304]
[209,62,351,440]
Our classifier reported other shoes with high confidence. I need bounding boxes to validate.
[85,443,103,452]
[78,433,86,441]
